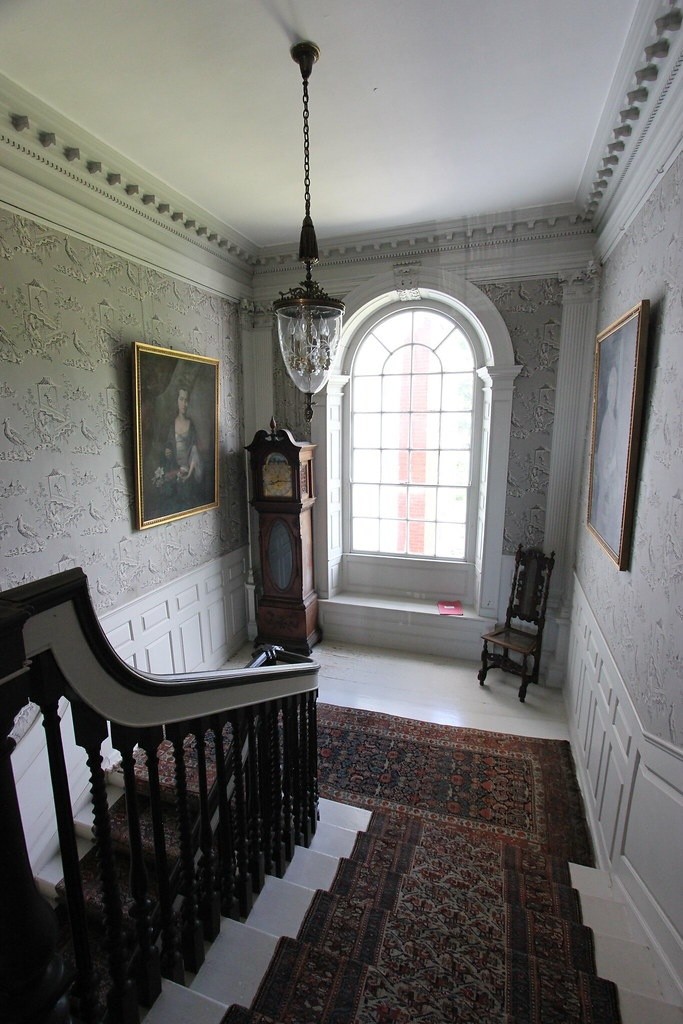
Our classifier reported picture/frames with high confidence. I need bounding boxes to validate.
[587,300,650,571]
[132,341,221,532]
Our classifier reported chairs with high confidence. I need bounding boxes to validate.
[477,543,556,703]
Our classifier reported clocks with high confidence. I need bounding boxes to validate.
[243,416,324,657]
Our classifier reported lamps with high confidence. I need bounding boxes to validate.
[271,40,346,421]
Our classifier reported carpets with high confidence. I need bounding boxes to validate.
[114,701,596,871]
[220,810,625,1024]
[47,778,205,1024]
